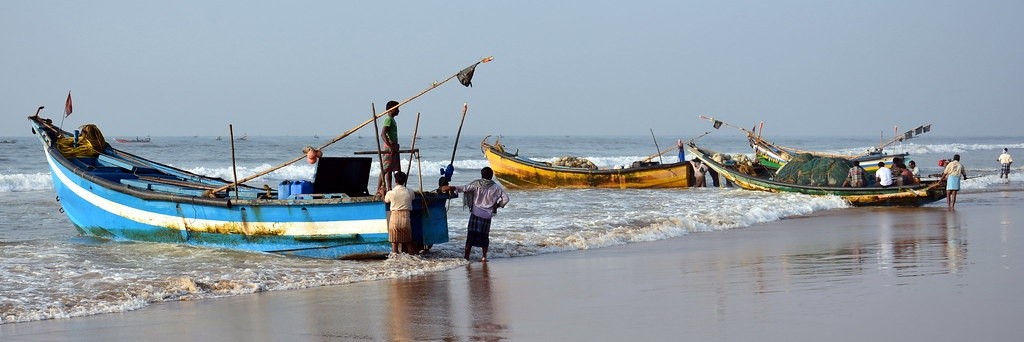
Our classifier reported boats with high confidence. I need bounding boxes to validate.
[480,134,707,188]
[688,116,956,205]
[27,105,459,262]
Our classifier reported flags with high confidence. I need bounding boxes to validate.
[64,94,73,115]
[904,130,913,140]
[922,125,931,133]
[915,125,923,135]
[712,120,723,128]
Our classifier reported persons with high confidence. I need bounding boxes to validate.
[996,147,1012,182]
[677,138,686,163]
[891,157,905,180]
[379,171,417,258]
[689,158,709,188]
[908,161,920,185]
[425,177,452,197]
[840,161,865,188]
[899,164,913,186]
[939,154,968,211]
[874,162,895,187]
[374,99,402,196]
[442,166,509,262]
